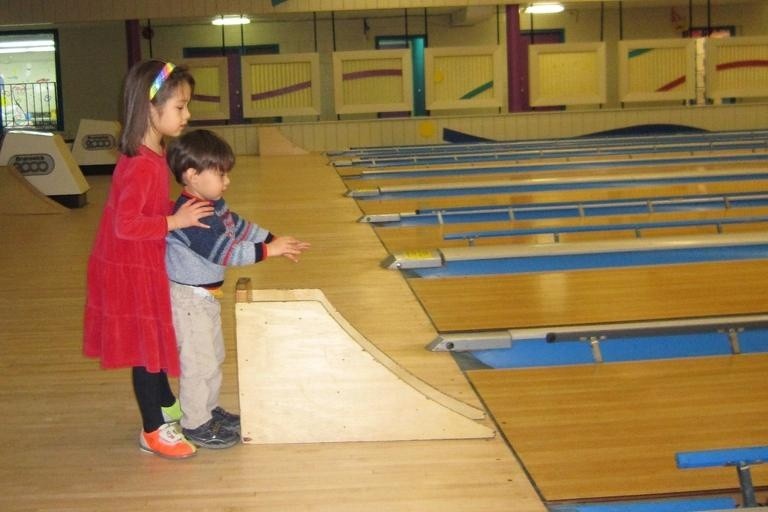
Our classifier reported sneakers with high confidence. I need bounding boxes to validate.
[161,398,183,423]
[182,407,240,448]
[139,422,197,459]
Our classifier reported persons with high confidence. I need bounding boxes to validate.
[162,128,313,450]
[81,57,218,461]
